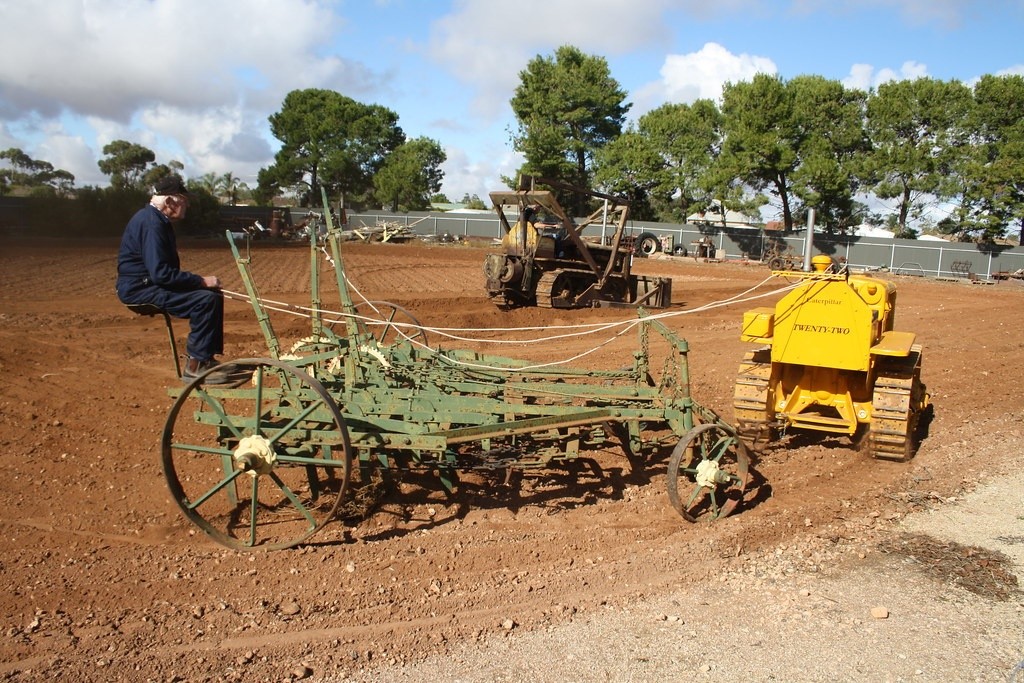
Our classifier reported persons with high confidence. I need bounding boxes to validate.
[116,177,239,384]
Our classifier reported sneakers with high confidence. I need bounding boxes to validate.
[206,356,238,373]
[179,354,229,383]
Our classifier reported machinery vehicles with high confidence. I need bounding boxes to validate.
[482,171,673,312]
[729,208,931,463]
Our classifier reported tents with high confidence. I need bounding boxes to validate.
[686,198,760,230]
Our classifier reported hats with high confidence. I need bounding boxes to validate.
[152,178,192,199]
